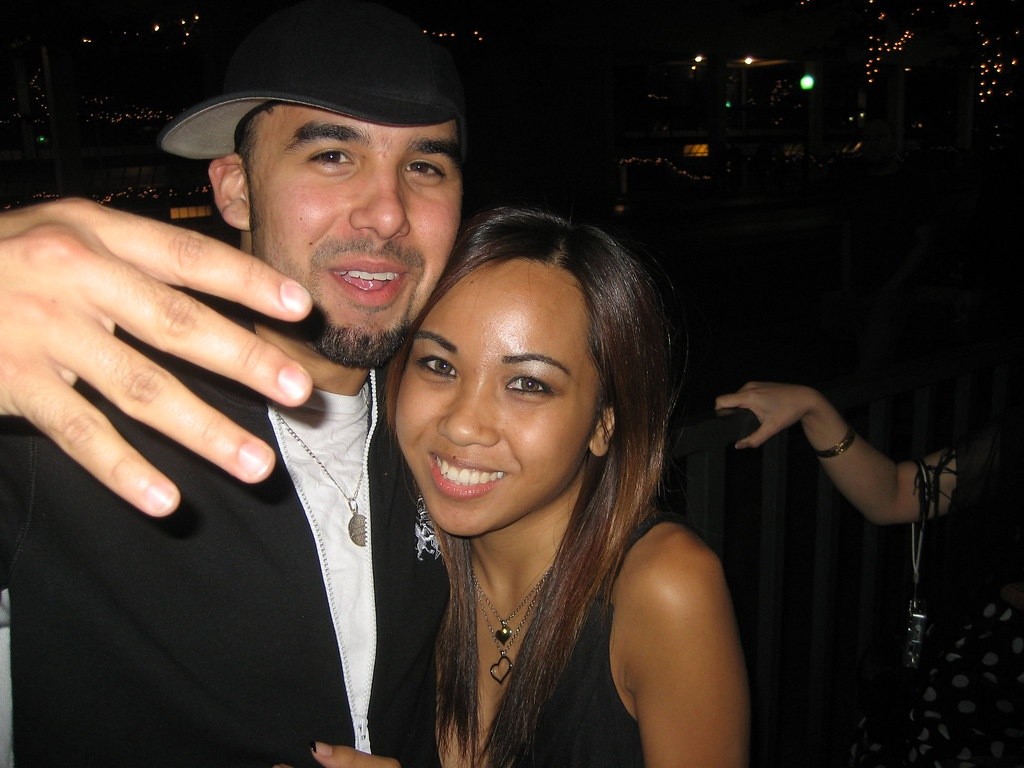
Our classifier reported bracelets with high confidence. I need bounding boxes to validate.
[814,423,855,458]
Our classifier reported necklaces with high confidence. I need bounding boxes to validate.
[268,385,370,546]
[470,562,554,685]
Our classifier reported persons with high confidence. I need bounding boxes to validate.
[716,381,1024,768]
[272,205,749,768]
[0,1,468,768]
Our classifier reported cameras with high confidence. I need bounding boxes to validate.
[903,599,928,669]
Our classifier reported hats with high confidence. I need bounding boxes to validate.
[155,0,468,165]
